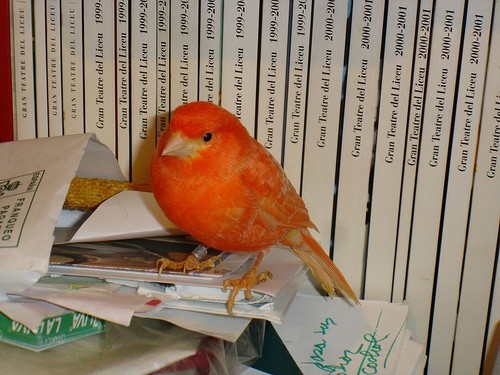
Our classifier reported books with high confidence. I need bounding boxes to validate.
[9,0,500,375]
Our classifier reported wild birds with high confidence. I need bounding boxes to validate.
[147,98,363,318]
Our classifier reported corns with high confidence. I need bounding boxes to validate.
[61,176,152,210]
